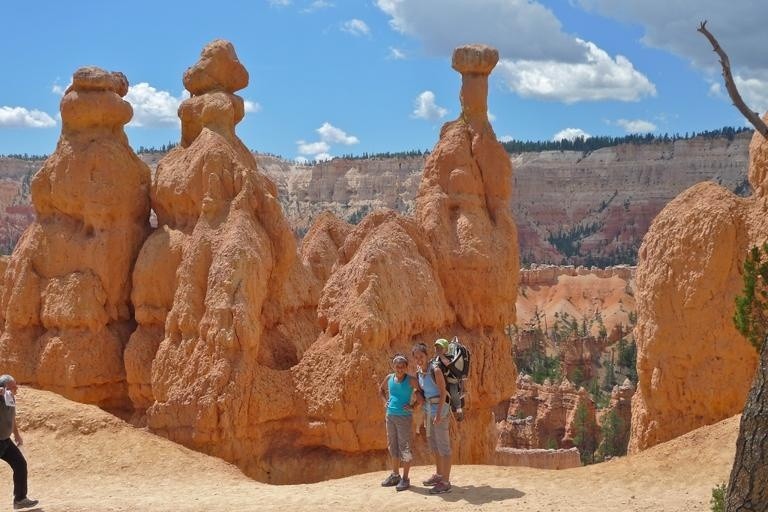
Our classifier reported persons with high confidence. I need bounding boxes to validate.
[0,373,39,510]
[433,338,464,423]
[378,353,425,491]
[410,342,453,494]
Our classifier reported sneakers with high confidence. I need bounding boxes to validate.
[13,497,39,512]
[423,474,443,487]
[396,477,411,491]
[428,479,452,494]
[457,408,464,422]
[381,472,402,487]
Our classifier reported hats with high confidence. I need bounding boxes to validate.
[434,338,449,349]
